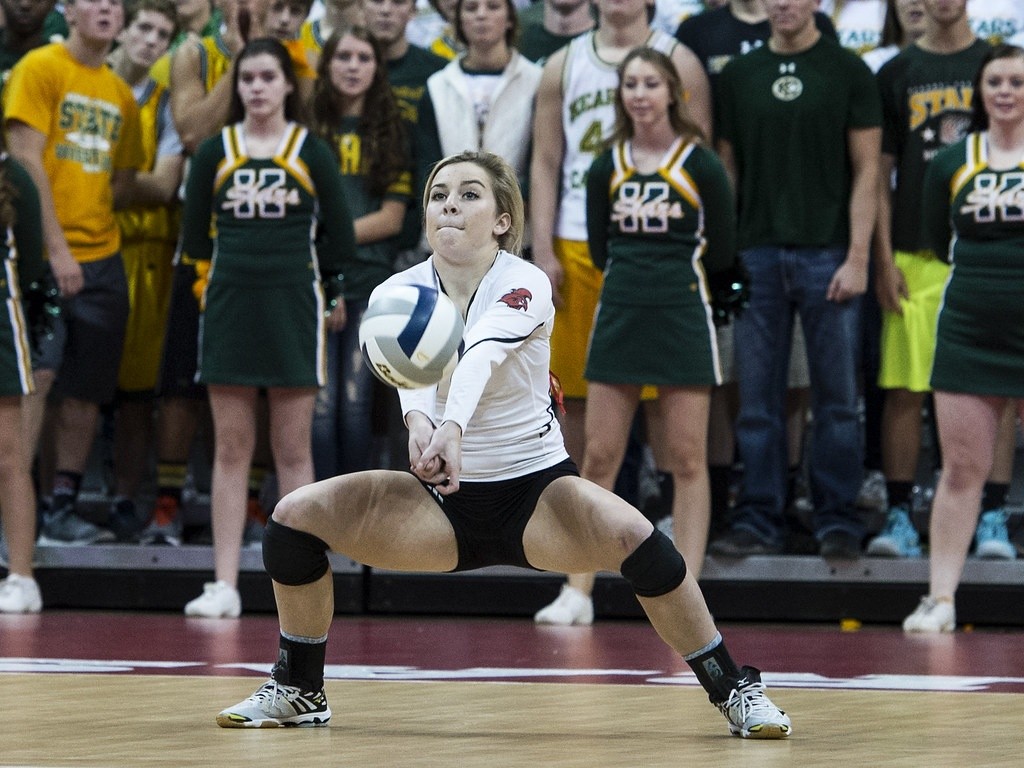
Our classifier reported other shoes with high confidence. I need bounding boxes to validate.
[709,524,785,555]
[821,528,863,556]
[37,506,115,546]
[136,496,184,548]
[240,497,270,548]
[857,470,888,514]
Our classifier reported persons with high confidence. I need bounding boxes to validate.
[172,34,354,617]
[535,48,734,625]
[215,151,792,740]
[0,0,1024,618]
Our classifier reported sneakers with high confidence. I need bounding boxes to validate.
[710,680,793,739]
[0,573,42,614]
[185,579,242,618]
[216,680,333,727]
[866,508,923,558]
[534,584,594,625]
[975,510,1017,562]
[902,593,955,633]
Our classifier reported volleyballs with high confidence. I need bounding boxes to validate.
[358,283,466,390]
